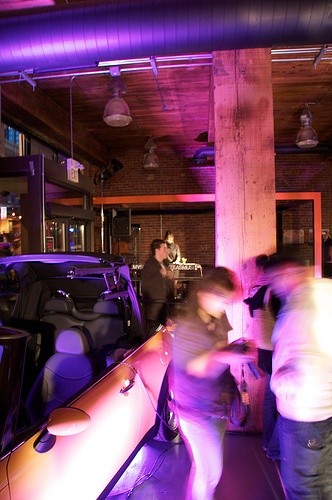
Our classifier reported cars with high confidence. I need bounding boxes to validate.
[0,251,174,500]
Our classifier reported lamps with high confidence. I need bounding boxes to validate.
[102,78,132,126]
[143,135,159,170]
[295,107,318,148]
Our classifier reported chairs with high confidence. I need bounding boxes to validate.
[40,288,124,350]
[25,329,95,425]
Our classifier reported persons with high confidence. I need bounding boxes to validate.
[171,266,256,500]
[244,249,332,500]
[162,231,182,299]
[139,239,175,339]
